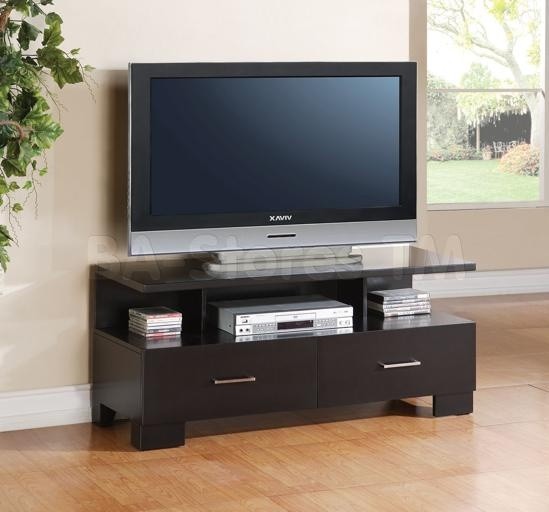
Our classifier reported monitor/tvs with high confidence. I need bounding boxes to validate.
[126,60,418,272]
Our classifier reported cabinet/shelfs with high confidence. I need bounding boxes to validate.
[90,245,477,452]
